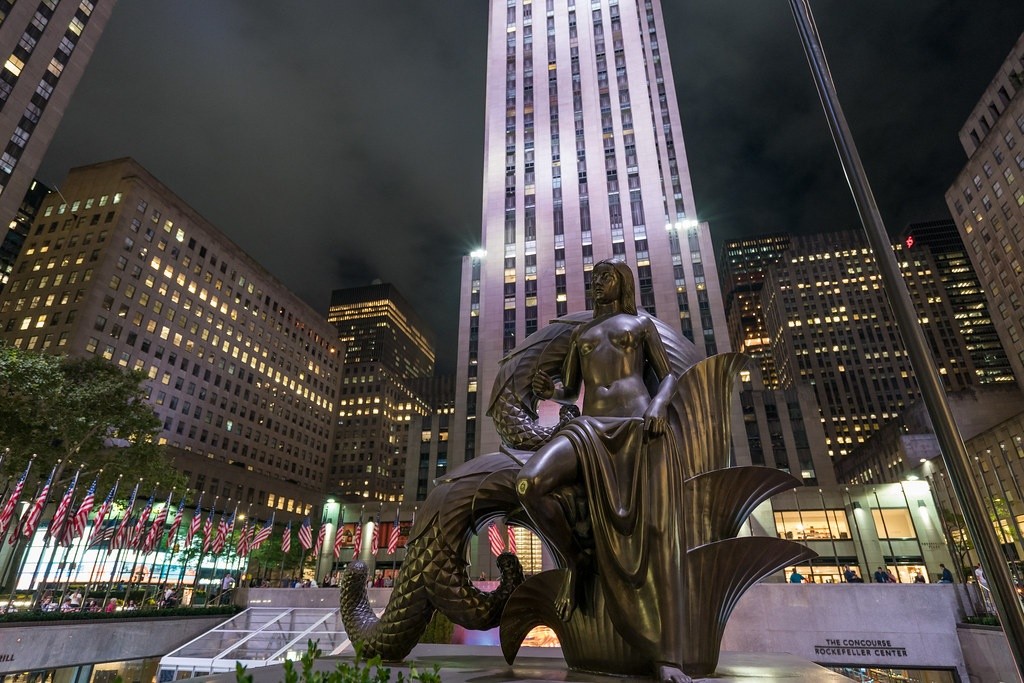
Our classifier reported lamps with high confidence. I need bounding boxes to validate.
[367,517,374,524]
[917,500,927,509]
[326,518,333,526]
[854,501,862,509]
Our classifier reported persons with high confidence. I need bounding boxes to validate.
[974,563,997,615]
[219,572,235,605]
[790,568,808,584]
[515,261,679,626]
[935,564,954,584]
[248,572,395,589]
[843,565,897,584]
[0,577,179,613]
[914,570,926,585]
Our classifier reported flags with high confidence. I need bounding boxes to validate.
[507,524,519,555]
[488,518,506,558]
[0,455,403,562]
